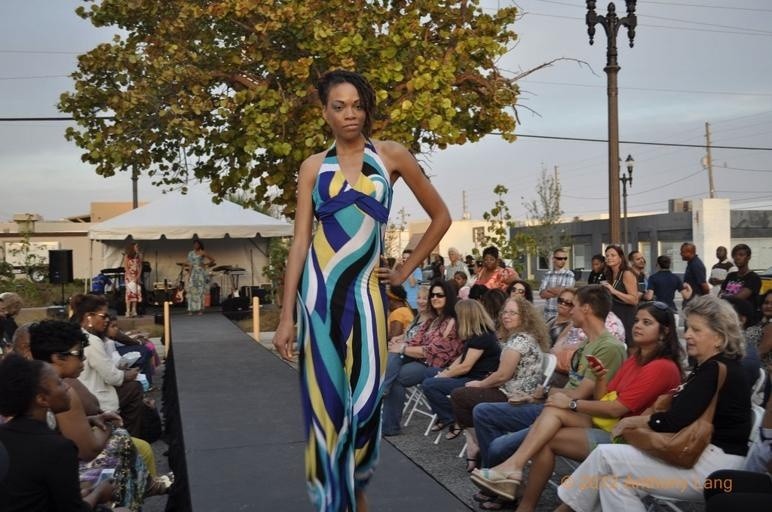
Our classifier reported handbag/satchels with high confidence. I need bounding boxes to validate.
[621,392,714,470]
[593,389,620,434]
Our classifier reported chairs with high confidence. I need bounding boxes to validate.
[646,368,766,512]
[400,336,558,459]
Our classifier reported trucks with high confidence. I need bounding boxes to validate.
[0,240,61,283]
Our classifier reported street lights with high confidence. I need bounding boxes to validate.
[584,0,639,262]
[614,154,634,262]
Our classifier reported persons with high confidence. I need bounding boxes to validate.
[125,243,142,318]
[182,240,214,317]
[270,68,452,511]
[1,292,174,512]
[381,241,772,511]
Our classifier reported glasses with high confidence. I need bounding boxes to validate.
[635,301,677,313]
[557,297,574,308]
[58,348,84,360]
[511,287,525,294]
[499,309,521,317]
[91,311,110,319]
[430,292,445,298]
[554,256,568,261]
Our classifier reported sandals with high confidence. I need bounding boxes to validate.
[471,468,522,499]
[160,472,174,493]
[446,424,462,439]
[466,450,481,472]
[431,421,447,432]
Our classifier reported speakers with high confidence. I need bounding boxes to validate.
[48,247,74,284]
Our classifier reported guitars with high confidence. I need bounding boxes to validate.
[171,265,186,306]
